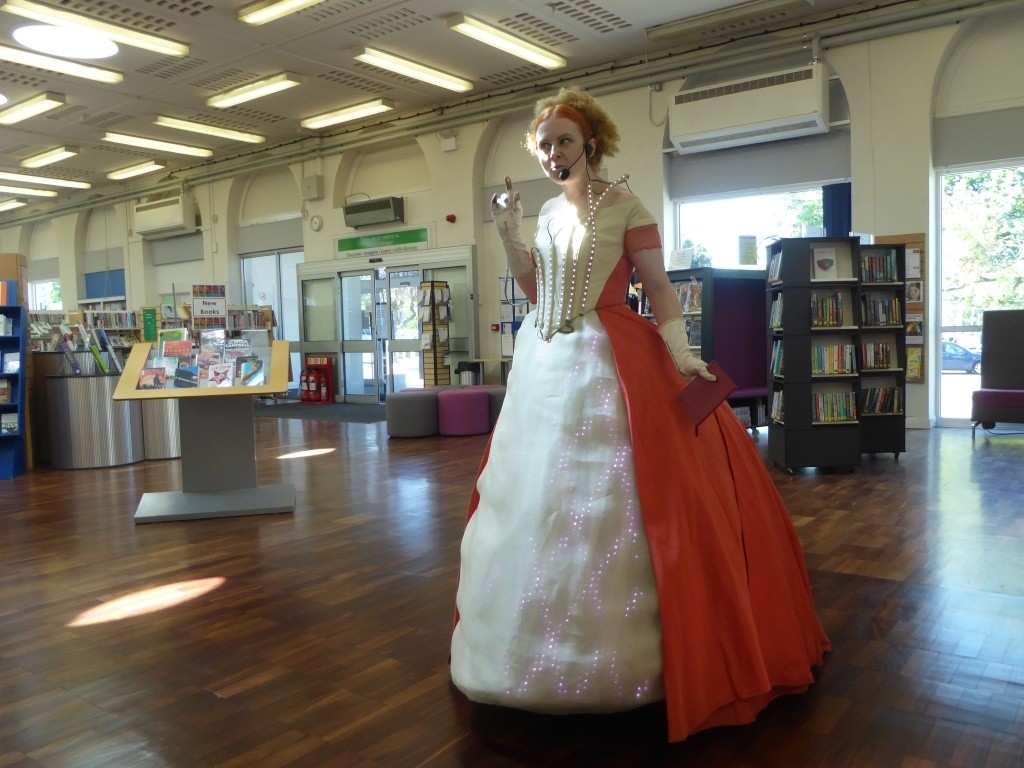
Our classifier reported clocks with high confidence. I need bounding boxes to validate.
[310,215,323,232]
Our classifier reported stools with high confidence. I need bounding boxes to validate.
[386,386,507,437]
[971,390,1024,440]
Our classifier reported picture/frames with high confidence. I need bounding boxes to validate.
[906,346,924,385]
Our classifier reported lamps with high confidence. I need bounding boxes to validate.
[0,0,568,211]
[646,0,817,44]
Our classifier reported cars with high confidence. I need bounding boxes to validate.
[942,340,982,374]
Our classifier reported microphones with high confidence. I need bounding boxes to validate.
[559,145,584,180]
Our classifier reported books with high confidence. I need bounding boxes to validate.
[815,386,905,422]
[810,246,899,284]
[0,313,20,435]
[643,279,701,350]
[767,251,784,426]
[229,306,270,329]
[809,292,901,327]
[905,247,926,384]
[28,309,138,352]
[811,341,894,374]
[139,328,265,389]
[160,299,192,326]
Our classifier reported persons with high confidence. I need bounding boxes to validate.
[906,323,921,337]
[908,282,921,302]
[448,89,826,743]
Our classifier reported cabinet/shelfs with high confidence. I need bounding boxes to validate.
[767,237,907,473]
[0,253,272,479]
[641,267,770,427]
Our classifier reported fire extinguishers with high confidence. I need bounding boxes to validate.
[308,369,320,401]
[300,369,309,401]
[319,373,328,401]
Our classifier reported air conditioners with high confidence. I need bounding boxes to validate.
[669,63,830,155]
[342,196,403,227]
[134,195,196,240]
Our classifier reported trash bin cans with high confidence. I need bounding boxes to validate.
[459,362,480,385]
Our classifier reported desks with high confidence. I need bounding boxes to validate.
[474,359,514,385]
